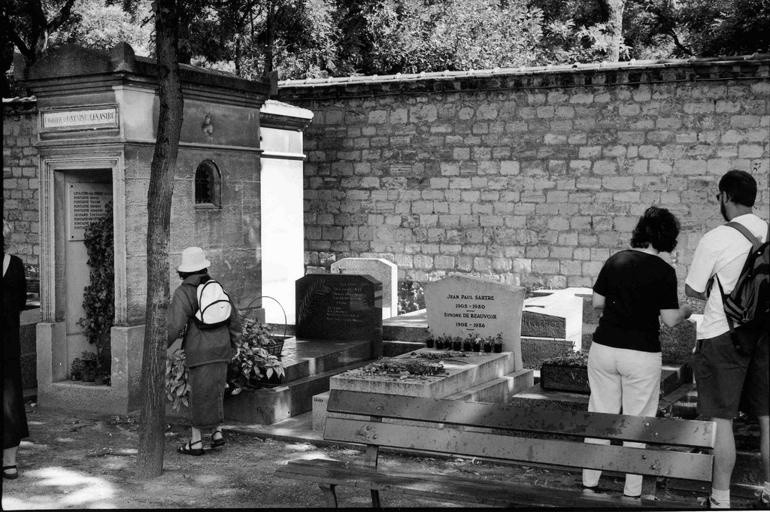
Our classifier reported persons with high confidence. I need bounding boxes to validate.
[581,206,696,500]
[166,246,243,457]
[2,219,28,479]
[684,168,769,509]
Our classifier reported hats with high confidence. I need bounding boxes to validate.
[177,247,211,273]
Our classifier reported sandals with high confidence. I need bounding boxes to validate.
[210,430,227,447]
[2,464,19,480]
[177,439,204,455]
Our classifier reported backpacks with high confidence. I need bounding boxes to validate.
[193,278,233,329]
[716,223,769,333]
